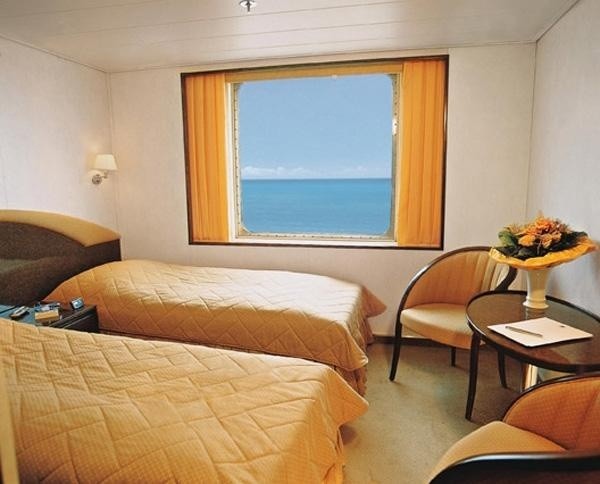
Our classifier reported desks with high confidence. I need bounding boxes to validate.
[465,290,599,420]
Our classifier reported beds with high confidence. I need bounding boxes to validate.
[0,318,370,484]
[43,260,388,397]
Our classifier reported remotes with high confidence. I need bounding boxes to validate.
[9,305,30,318]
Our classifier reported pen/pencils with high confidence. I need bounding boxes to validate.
[506,326,542,338]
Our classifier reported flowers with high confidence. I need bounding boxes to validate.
[497,210,586,261]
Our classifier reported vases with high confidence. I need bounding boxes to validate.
[522,270,551,309]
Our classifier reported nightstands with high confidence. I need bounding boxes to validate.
[0,301,100,333]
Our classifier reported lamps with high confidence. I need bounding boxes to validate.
[90,154,118,185]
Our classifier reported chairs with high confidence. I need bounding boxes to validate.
[428,372,600,484]
[389,246,519,422]
